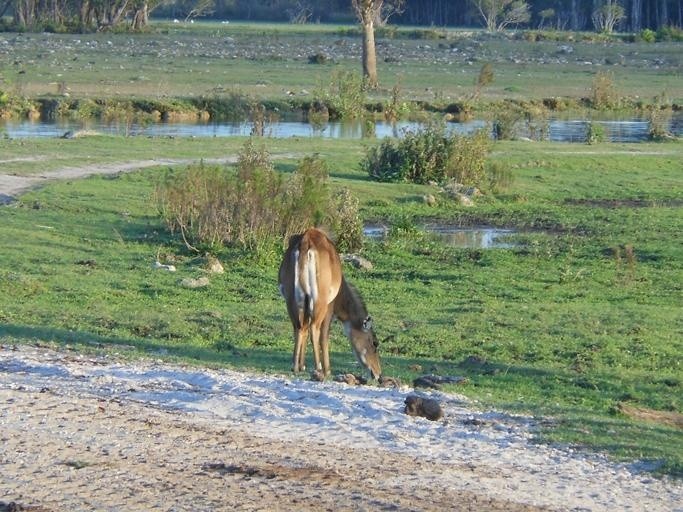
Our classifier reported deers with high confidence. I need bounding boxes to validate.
[277,228,382,382]
